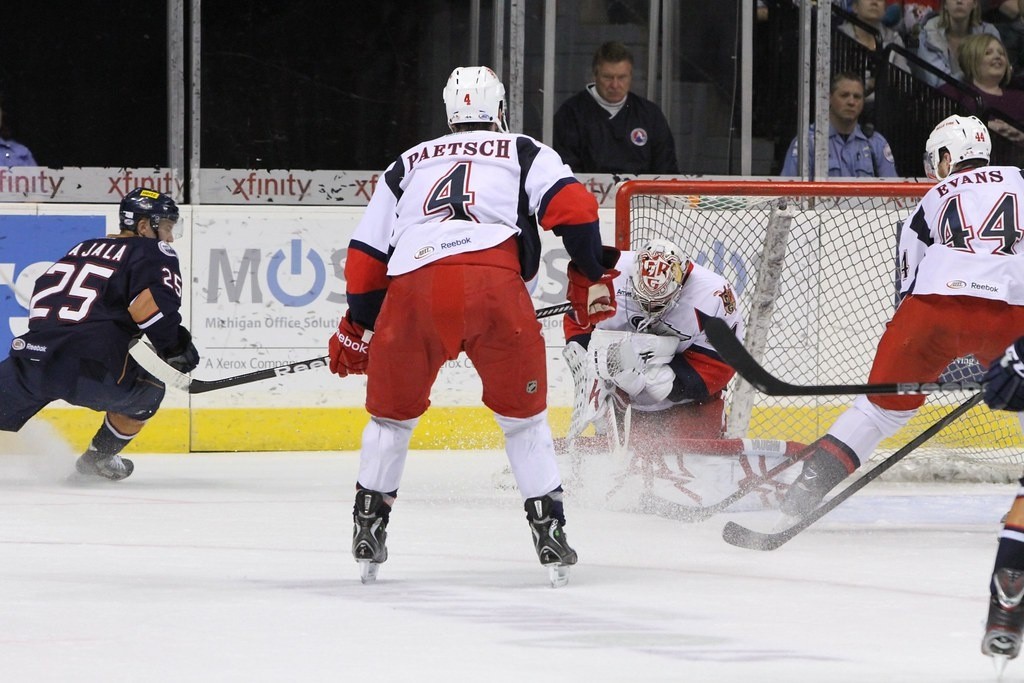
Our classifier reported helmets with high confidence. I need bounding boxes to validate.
[633,239,683,301]
[925,116,992,177]
[442,63,505,127]
[121,188,180,232]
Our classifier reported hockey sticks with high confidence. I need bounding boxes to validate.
[127,302,576,396]
[640,435,819,523]
[722,391,986,552]
[604,398,622,482]
[703,315,988,397]
[0,266,157,355]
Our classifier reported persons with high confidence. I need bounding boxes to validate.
[553,42,679,174]
[780,69,898,177]
[0,188,200,480]
[779,110,1024,662]
[328,68,616,561]
[563,237,743,440]
[831,0,1024,148]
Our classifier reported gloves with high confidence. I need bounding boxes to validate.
[567,244,622,330]
[158,324,201,372]
[981,334,1024,411]
[328,307,373,379]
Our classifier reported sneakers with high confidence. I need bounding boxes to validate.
[69,437,134,479]
[775,458,837,522]
[524,487,577,586]
[352,482,399,585]
[980,567,1024,672]
